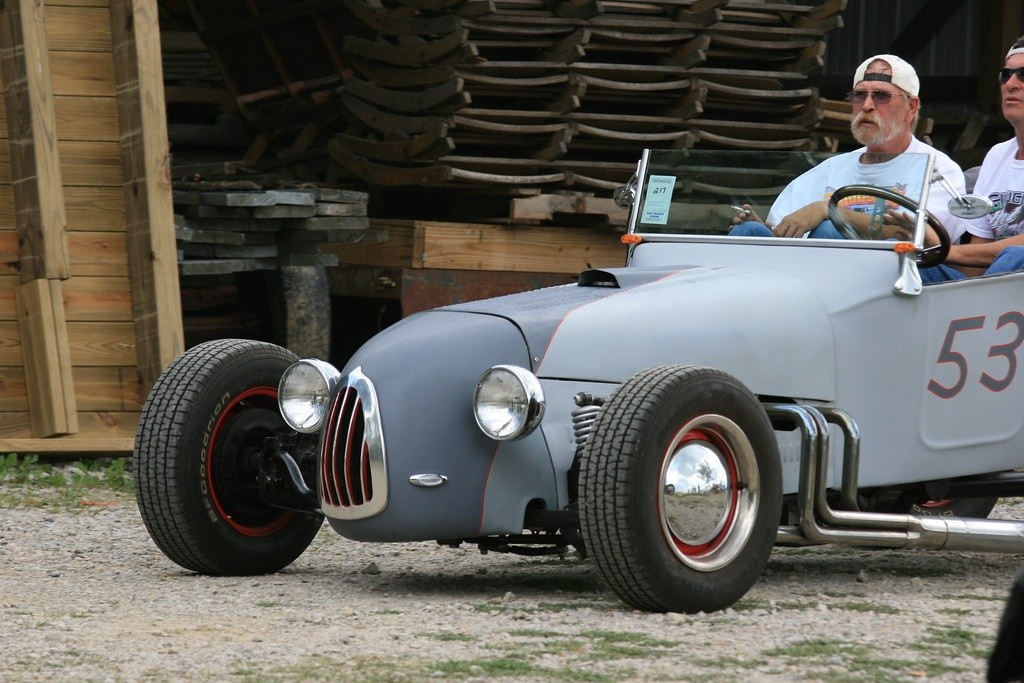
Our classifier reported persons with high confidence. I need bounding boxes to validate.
[883,38,1024,284]
[728,55,968,240]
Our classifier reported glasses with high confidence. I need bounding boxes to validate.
[991,68,1024,92]
[847,90,904,105]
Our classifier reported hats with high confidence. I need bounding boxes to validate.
[1004,34,1024,62]
[852,54,920,97]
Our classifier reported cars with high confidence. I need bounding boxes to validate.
[131,147,1023,614]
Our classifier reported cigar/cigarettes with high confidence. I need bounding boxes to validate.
[731,205,750,215]
